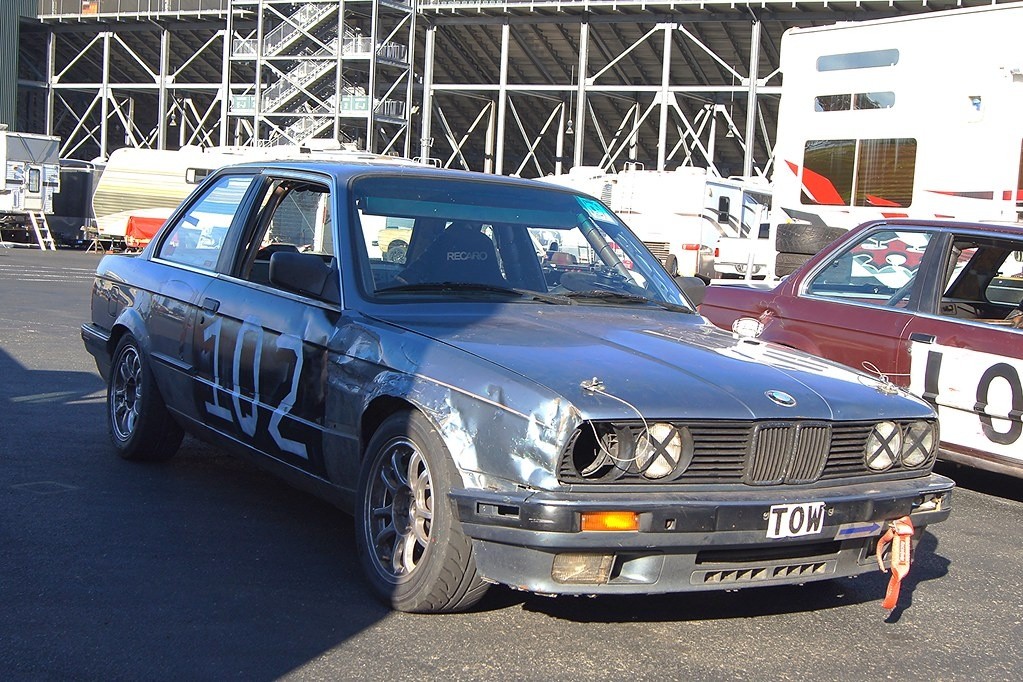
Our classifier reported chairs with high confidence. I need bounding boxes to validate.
[429,230,511,288]
[270,252,339,303]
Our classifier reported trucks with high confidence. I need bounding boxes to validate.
[608,161,762,284]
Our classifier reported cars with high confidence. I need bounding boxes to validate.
[374,218,415,264]
[76,156,960,615]
[698,215,1023,482]
[713,220,769,279]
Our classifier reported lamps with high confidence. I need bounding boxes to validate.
[726,65,736,138]
[566,64,574,134]
